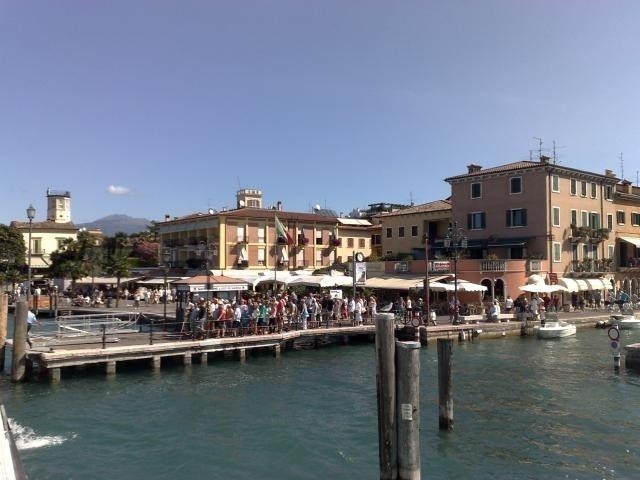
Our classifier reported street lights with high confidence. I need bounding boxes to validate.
[25,203,37,312]
[162,262,172,332]
[205,258,214,338]
[444,220,469,326]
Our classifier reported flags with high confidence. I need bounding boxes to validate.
[275,214,294,246]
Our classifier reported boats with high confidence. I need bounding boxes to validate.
[608,313,640,330]
[531,317,577,340]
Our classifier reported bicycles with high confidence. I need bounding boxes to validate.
[400,308,420,327]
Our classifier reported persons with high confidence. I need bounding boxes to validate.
[1,281,179,309]
[487,293,560,324]
[570,288,640,313]
[183,288,471,339]
[26,305,41,349]
